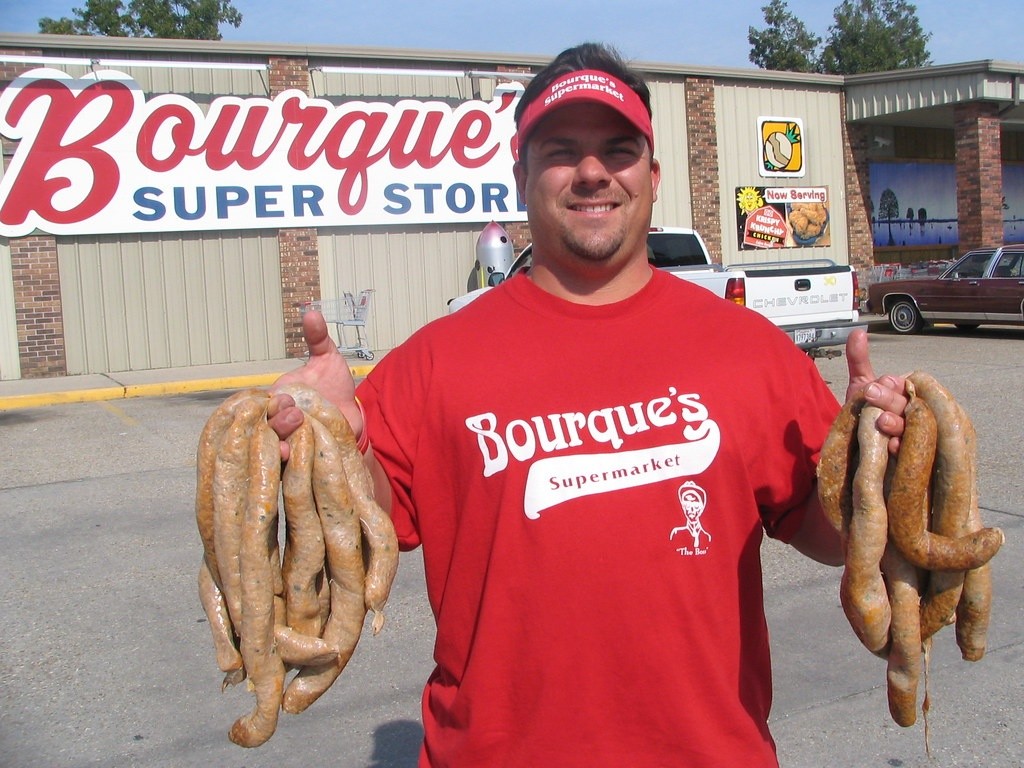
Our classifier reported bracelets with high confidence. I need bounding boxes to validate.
[355,396,368,451]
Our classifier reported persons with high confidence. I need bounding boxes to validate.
[267,42,908,768]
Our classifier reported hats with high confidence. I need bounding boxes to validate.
[518,69,654,164]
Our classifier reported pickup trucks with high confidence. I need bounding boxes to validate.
[870,243,1024,337]
[444,224,869,372]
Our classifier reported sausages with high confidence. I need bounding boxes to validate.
[196,379,399,748]
[815,371,1004,727]
